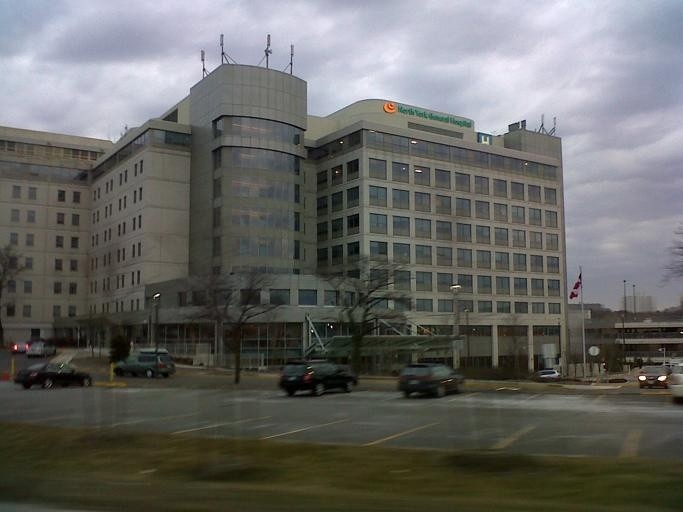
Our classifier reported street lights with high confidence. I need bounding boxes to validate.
[449,283,462,335]
[658,347,665,364]
[151,292,162,379]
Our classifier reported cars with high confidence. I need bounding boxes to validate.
[15,360,94,391]
[11,336,57,358]
[667,363,682,398]
[395,361,466,398]
[113,348,176,380]
[637,364,672,389]
[278,356,360,396]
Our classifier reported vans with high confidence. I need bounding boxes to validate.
[537,369,559,379]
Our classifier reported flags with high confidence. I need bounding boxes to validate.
[567,274,581,299]
